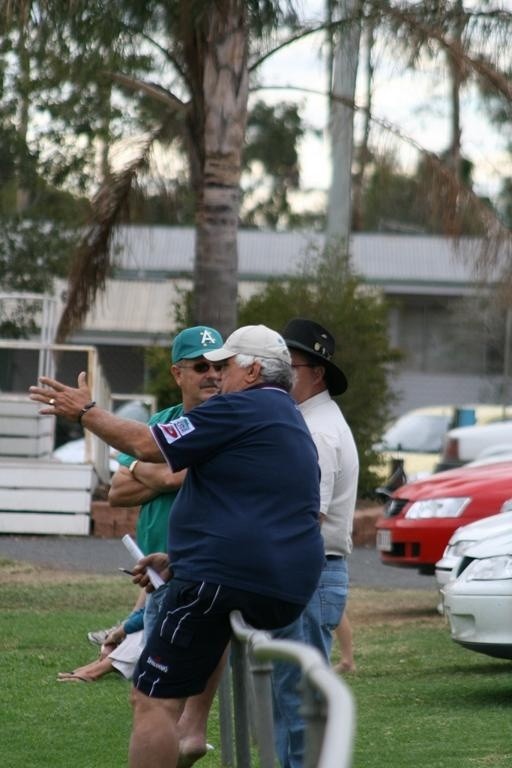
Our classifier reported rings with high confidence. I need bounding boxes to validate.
[48,396,56,406]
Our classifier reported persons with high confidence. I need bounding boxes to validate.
[87,586,151,648]
[106,323,237,768]
[265,323,363,768]
[28,323,329,768]
[57,642,142,685]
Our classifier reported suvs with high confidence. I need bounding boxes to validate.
[375,422,512,507]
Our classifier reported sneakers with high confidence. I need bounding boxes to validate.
[87,627,125,646]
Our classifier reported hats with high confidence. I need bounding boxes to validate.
[171,326,223,364]
[203,324,292,365]
[282,318,348,397]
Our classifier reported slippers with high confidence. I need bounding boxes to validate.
[56,672,75,677]
[56,675,89,684]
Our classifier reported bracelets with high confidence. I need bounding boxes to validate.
[77,401,95,427]
[128,459,141,480]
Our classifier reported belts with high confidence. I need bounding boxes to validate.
[324,554,344,562]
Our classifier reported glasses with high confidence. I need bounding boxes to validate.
[183,363,223,373]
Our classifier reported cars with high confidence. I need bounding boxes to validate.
[369,404,512,476]
[375,458,512,572]
[437,537,512,664]
[433,509,512,619]
